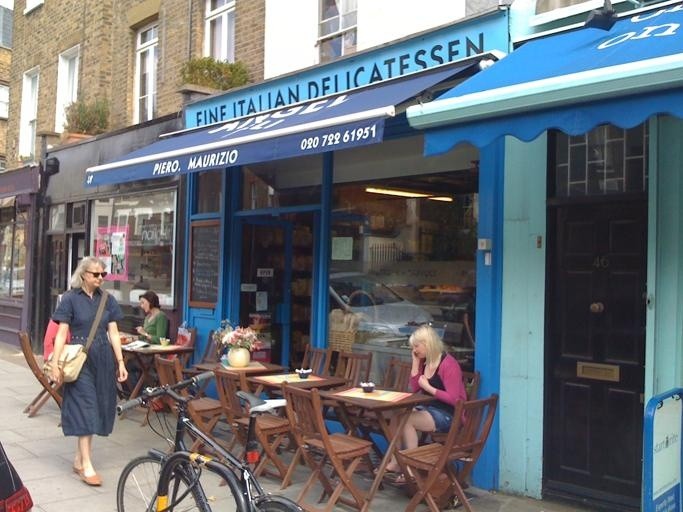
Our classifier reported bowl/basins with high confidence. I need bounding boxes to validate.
[160,339,171,346]
[298,373,310,379]
[362,387,374,393]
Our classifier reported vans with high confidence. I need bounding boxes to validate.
[326,270,434,348]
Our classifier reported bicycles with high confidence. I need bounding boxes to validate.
[114,368,311,512]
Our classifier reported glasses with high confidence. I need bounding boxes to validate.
[82,270,107,278]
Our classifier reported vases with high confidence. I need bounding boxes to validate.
[227,347,250,369]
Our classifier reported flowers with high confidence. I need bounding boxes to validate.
[211,320,265,361]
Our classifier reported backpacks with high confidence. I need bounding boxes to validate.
[44,290,71,383]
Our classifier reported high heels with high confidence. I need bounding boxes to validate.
[72,455,80,472]
[76,461,101,486]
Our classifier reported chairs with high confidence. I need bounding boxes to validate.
[214,369,293,486]
[347,360,412,458]
[397,393,498,512]
[18,332,63,417]
[280,383,372,510]
[323,350,372,420]
[430,372,479,441]
[155,354,222,452]
[176,327,196,368]
[302,344,332,374]
[182,330,216,398]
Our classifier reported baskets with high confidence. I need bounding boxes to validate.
[330,314,368,352]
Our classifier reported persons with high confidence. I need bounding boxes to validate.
[43,316,71,366]
[373,325,467,486]
[51,257,128,485]
[125,292,169,398]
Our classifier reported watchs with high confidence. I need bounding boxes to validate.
[143,332,148,339]
